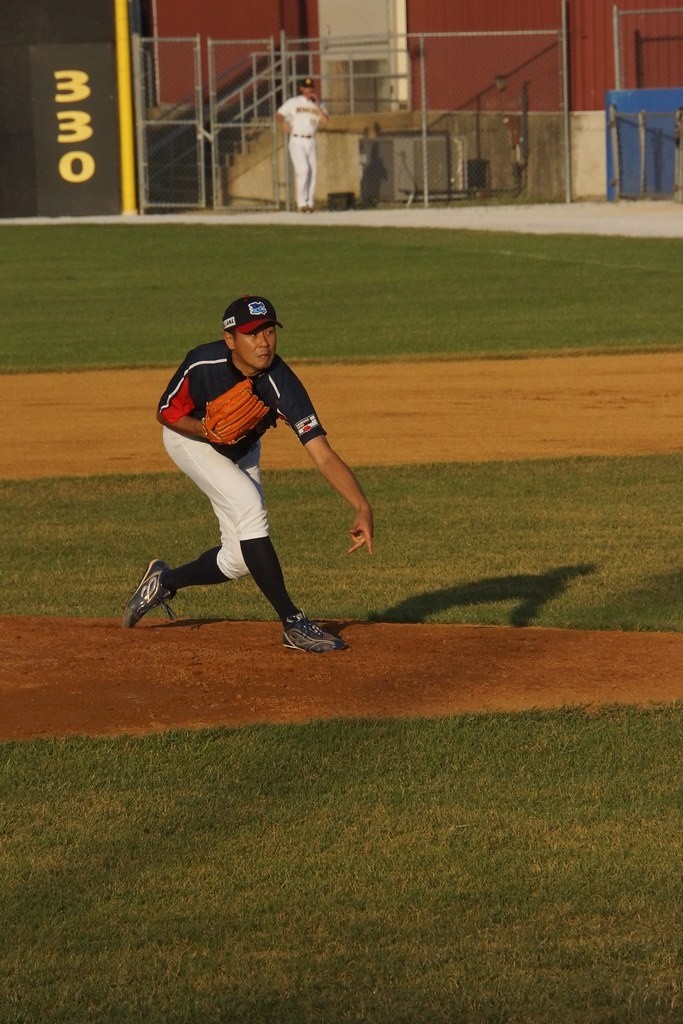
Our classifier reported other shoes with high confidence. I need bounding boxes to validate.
[297,207,313,213]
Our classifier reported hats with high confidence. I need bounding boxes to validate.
[223,294,284,333]
[300,77,314,88]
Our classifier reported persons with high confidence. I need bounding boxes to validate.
[123,293,374,654]
[276,77,329,213]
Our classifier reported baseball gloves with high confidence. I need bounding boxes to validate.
[202,377,270,446]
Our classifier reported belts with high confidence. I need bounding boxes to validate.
[292,134,311,139]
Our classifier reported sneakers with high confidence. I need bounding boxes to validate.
[121,558,180,629]
[281,607,350,653]
